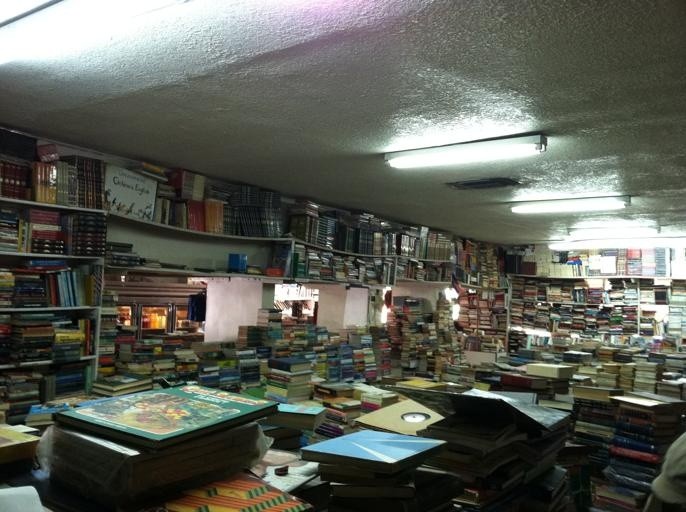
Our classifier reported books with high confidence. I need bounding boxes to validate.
[0,127,686,512]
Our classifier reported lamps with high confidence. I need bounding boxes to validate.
[511,195,631,214]
[385,132,547,168]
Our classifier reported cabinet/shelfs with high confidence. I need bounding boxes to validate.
[105,214,294,279]
[289,240,396,283]
[507,275,639,352]
[0,253,102,310]
[1,309,100,369]
[0,359,97,425]
[478,288,506,353]
[100,283,209,341]
[0,201,110,259]
[396,256,454,282]
[0,128,109,212]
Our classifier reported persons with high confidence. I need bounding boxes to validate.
[650,431,685,512]
